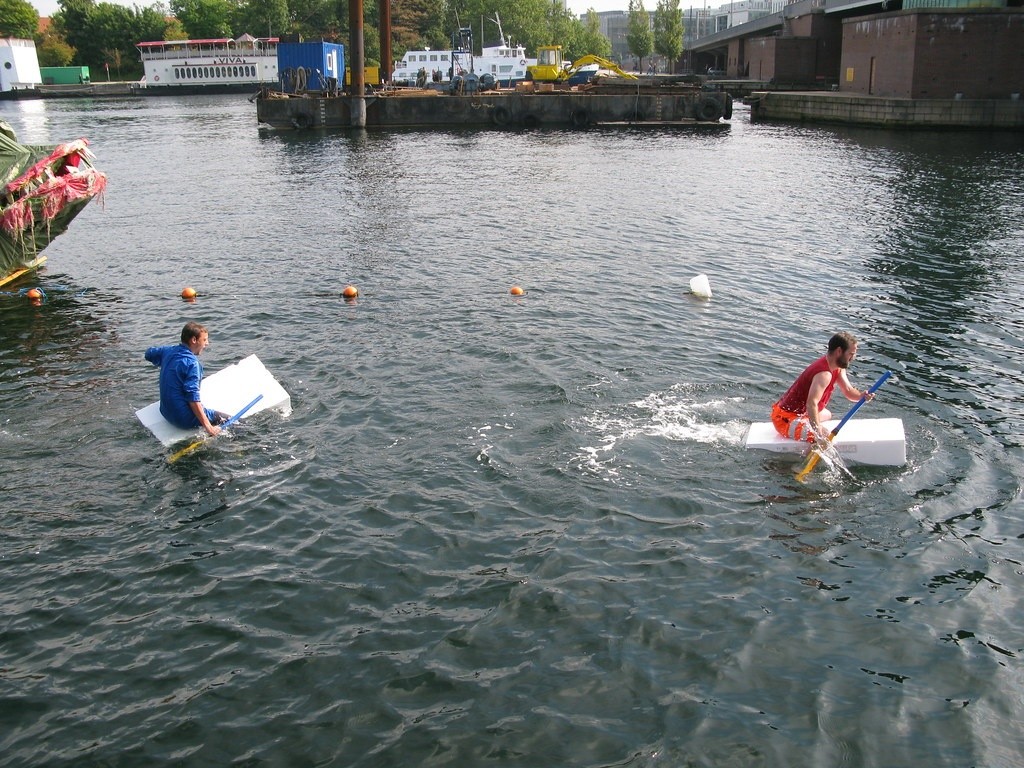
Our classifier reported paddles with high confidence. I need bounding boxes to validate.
[170,393,264,463]
[792,369,893,480]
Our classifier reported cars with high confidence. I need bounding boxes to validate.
[708,66,727,75]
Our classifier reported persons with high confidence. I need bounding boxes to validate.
[772,332,876,443]
[144,322,246,437]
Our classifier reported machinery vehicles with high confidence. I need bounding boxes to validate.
[527,44,639,90]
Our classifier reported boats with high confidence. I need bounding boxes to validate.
[131,33,280,95]
[392,34,527,88]
